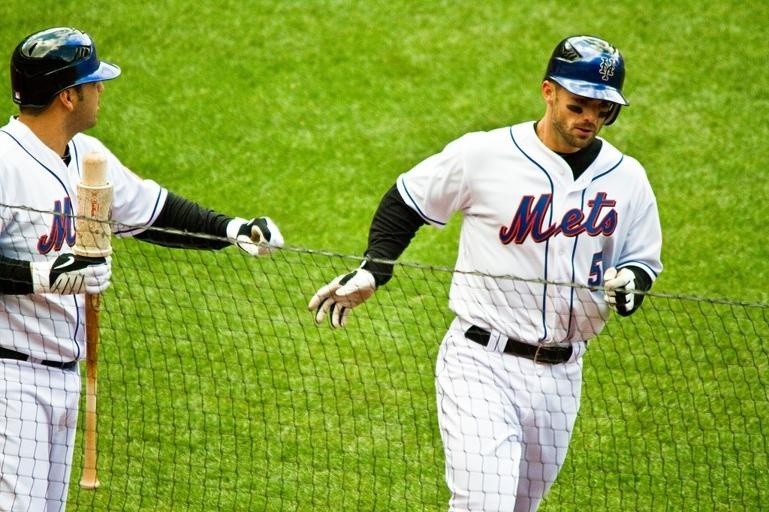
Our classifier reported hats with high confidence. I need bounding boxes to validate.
[11,27,122,109]
[543,35,630,106]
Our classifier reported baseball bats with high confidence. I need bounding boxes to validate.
[74,154,114,491]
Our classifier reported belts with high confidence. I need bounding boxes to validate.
[464,324,573,365]
[0,347,75,369]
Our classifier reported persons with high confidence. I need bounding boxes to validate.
[0,27,283,512]
[306,34,665,512]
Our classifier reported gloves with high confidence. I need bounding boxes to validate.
[226,216,285,258]
[30,253,113,295]
[308,268,375,329]
[603,265,651,317]
